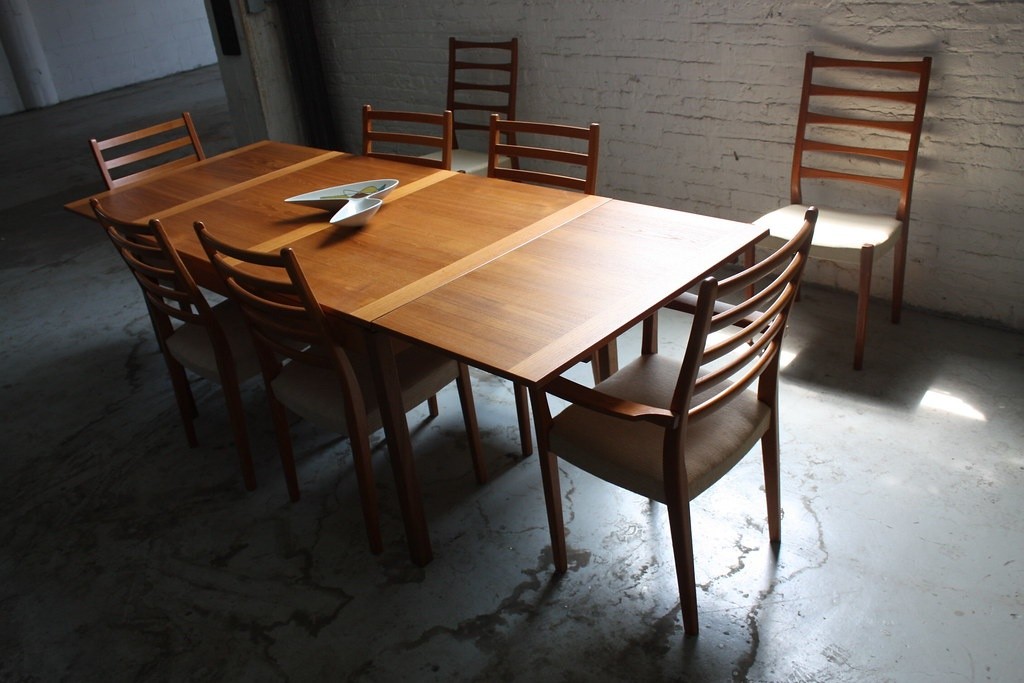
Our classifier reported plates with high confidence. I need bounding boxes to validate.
[283,178,400,227]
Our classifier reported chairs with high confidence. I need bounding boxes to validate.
[738,48,932,373]
[418,35,519,178]
[192,219,488,552]
[87,195,312,494]
[424,111,626,459]
[359,103,454,170]
[88,110,207,191]
[63,138,771,572]
[529,206,821,640]
